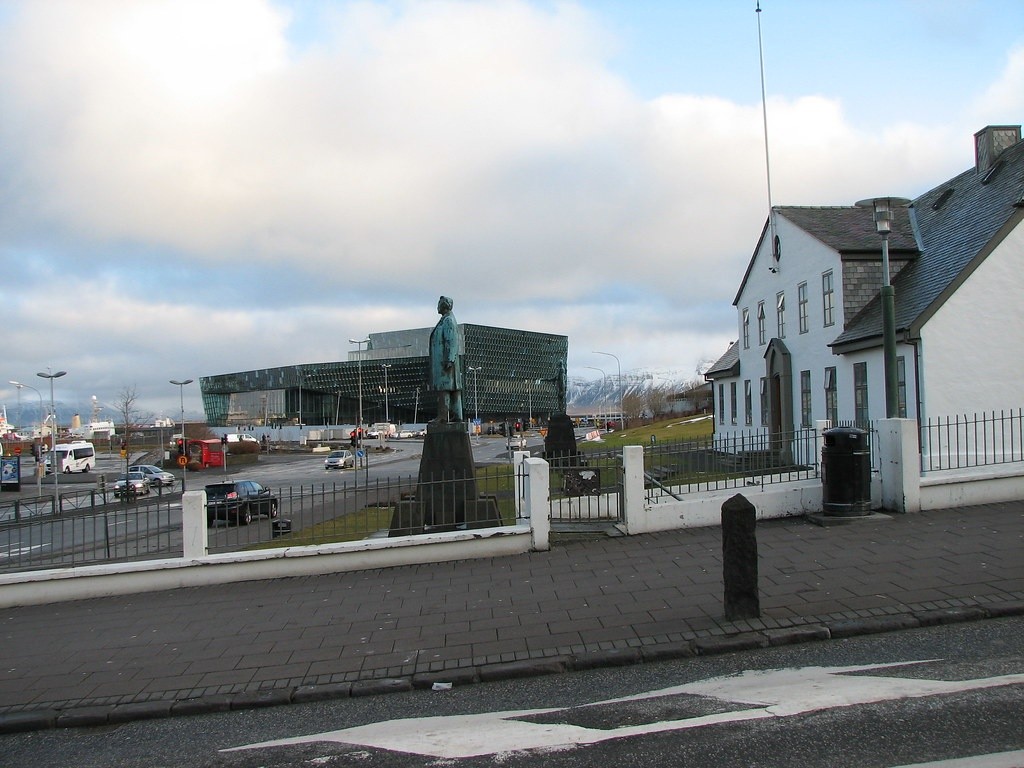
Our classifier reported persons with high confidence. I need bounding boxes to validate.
[365,430,367,436]
[243,424,246,431]
[262,435,266,444]
[121,444,126,450]
[427,296,463,423]
[123,440,126,446]
[248,424,251,430]
[238,424,241,434]
[239,435,243,442]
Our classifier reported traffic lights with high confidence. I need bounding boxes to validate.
[29,443,45,456]
[350,428,361,448]
[499,422,520,436]
[178,438,183,452]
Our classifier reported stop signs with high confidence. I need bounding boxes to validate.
[14,447,21,455]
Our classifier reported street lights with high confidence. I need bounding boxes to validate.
[349,338,420,467]
[524,379,539,427]
[8,370,194,498]
[593,352,625,430]
[585,366,607,433]
[856,195,912,419]
[299,375,342,435]
[468,365,483,443]
[658,378,674,417]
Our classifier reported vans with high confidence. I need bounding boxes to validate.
[44,440,96,474]
[228,434,261,444]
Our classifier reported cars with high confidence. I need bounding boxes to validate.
[368,426,427,439]
[114,463,176,498]
[506,436,527,450]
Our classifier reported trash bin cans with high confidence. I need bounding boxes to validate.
[271,519,292,539]
[820,426,872,517]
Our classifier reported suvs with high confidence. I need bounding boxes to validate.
[206,479,279,524]
